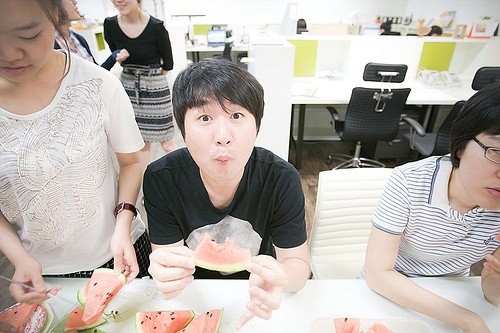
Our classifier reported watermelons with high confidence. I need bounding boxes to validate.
[135,307,224,333]
[64,267,127,331]
[0,302,37,333]
[333,317,393,333]
[192,232,251,272]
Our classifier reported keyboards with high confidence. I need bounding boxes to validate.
[207,44,225,47]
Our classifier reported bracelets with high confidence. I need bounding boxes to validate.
[114,202,138,223]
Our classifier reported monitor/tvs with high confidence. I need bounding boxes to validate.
[208,30,225,42]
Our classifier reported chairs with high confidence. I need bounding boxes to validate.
[307,168,395,280]
[324,62,411,172]
[400,66,500,161]
[214,31,235,61]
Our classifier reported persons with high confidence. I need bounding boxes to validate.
[425,26,443,37]
[378,22,391,34]
[364,81,500,333]
[0,0,152,305]
[296,19,309,35]
[141,58,311,330]
[54,0,130,71]
[103,0,178,205]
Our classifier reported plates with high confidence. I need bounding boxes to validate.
[0,276,500,333]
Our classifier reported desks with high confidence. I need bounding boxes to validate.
[288,77,478,170]
[43,273,500,333]
[186,46,249,63]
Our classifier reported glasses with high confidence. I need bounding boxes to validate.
[472,137,500,164]
